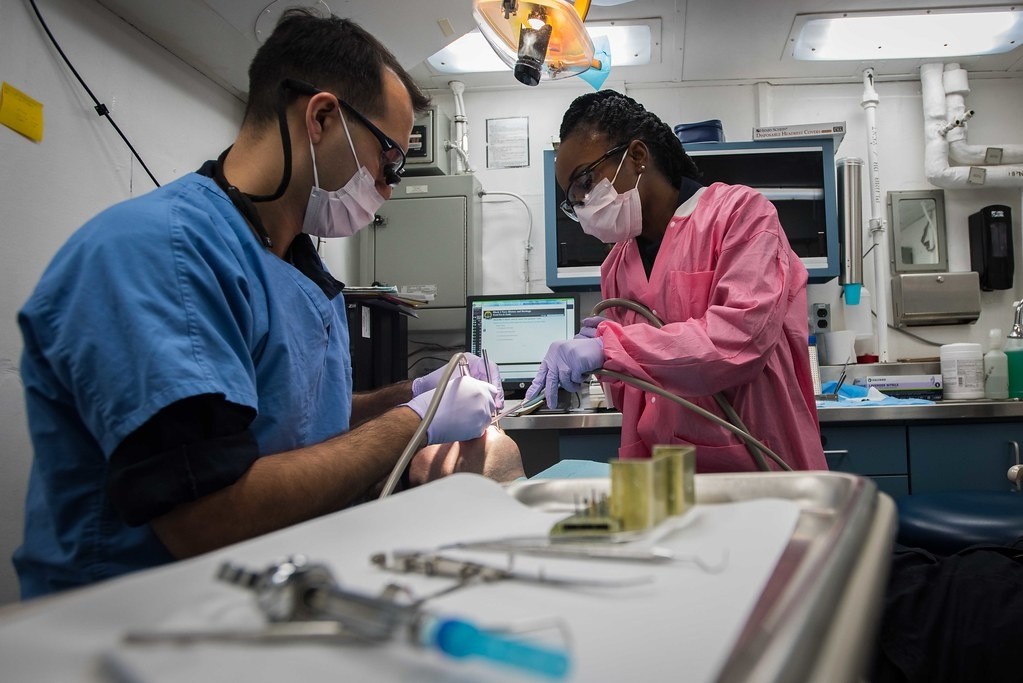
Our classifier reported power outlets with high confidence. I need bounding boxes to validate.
[813,303,831,334]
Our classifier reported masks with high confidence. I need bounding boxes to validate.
[303,107,385,239]
[573,148,642,243]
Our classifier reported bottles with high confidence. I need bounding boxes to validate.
[983,327,1009,400]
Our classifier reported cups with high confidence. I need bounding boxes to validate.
[825,330,857,366]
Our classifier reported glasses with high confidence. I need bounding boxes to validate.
[560,143,629,222]
[281,77,407,187]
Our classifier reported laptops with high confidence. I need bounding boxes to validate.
[465,291,581,413]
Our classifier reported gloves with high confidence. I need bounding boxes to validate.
[396,352,504,446]
[525,316,606,410]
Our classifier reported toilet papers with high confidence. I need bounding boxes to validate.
[824,331,858,365]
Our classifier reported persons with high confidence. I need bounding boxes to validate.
[10,9,504,602]
[404,425,1023,683]
[522,90,829,474]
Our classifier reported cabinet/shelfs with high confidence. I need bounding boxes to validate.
[360,174,483,332]
